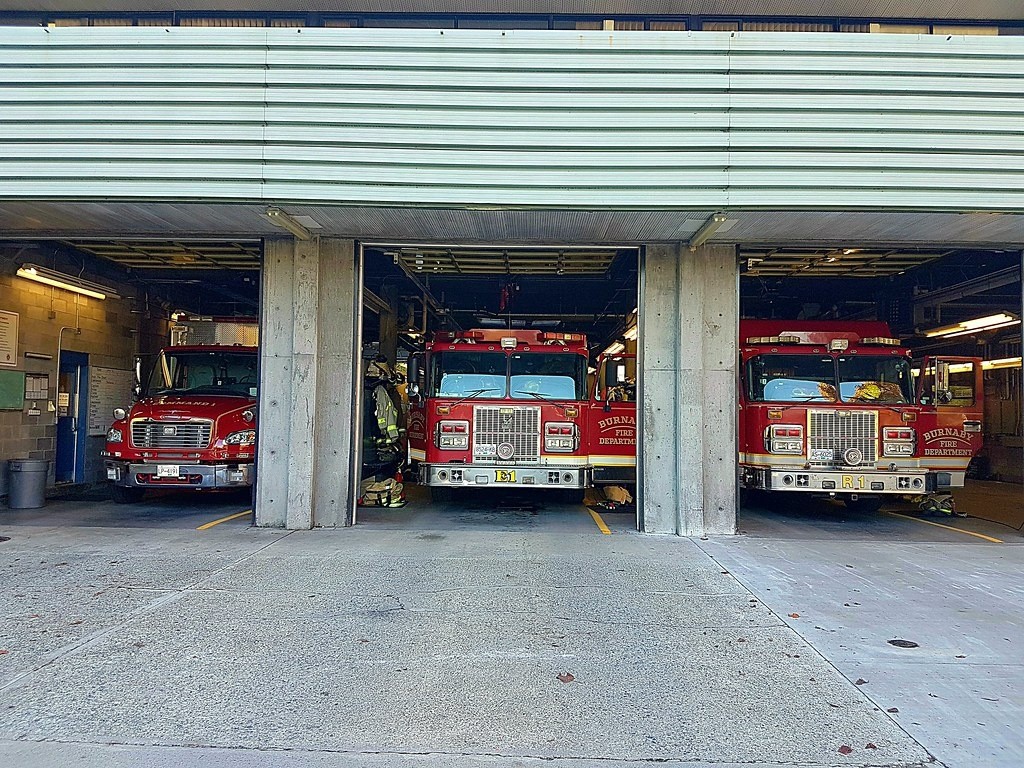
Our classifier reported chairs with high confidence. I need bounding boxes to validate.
[186,365,257,388]
[440,374,576,400]
[763,379,907,404]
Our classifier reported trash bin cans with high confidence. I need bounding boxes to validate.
[7,457,53,510]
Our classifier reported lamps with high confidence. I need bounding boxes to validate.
[266,207,312,242]
[688,214,728,247]
[923,310,1022,339]
[15,262,121,301]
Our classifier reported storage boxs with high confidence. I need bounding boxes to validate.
[931,385,974,406]
[984,400,1024,447]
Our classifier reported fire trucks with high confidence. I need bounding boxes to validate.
[407,328,637,508]
[740,320,984,513]
[96,314,262,503]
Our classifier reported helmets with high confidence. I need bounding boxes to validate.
[368,359,389,374]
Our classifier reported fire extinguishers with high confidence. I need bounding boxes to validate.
[394,467,405,499]
